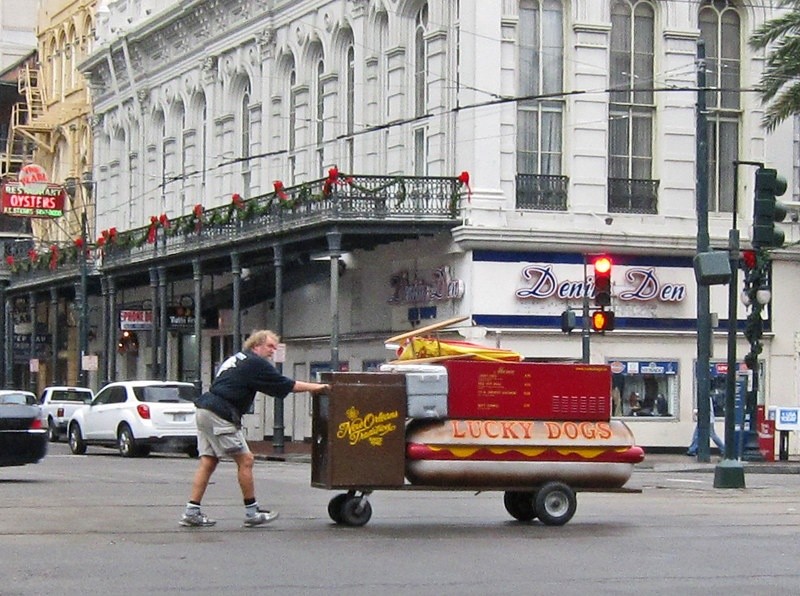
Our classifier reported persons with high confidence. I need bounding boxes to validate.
[688,396,724,456]
[655,394,668,416]
[179,329,330,527]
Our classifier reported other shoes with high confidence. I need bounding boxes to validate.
[686,451,696,456]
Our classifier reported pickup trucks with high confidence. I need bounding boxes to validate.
[37,388,94,442]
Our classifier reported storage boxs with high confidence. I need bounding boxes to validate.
[378,364,449,421]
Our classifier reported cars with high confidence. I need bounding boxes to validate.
[67,379,200,459]
[0,404,48,462]
[0,390,36,405]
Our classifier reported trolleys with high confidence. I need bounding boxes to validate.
[313,370,643,527]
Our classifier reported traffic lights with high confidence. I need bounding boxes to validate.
[752,167,787,245]
[593,311,615,331]
[594,256,612,306]
[561,311,575,332]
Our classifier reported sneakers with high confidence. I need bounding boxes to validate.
[243,509,279,526]
[179,513,216,526]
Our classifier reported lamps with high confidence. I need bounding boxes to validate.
[88,325,97,340]
